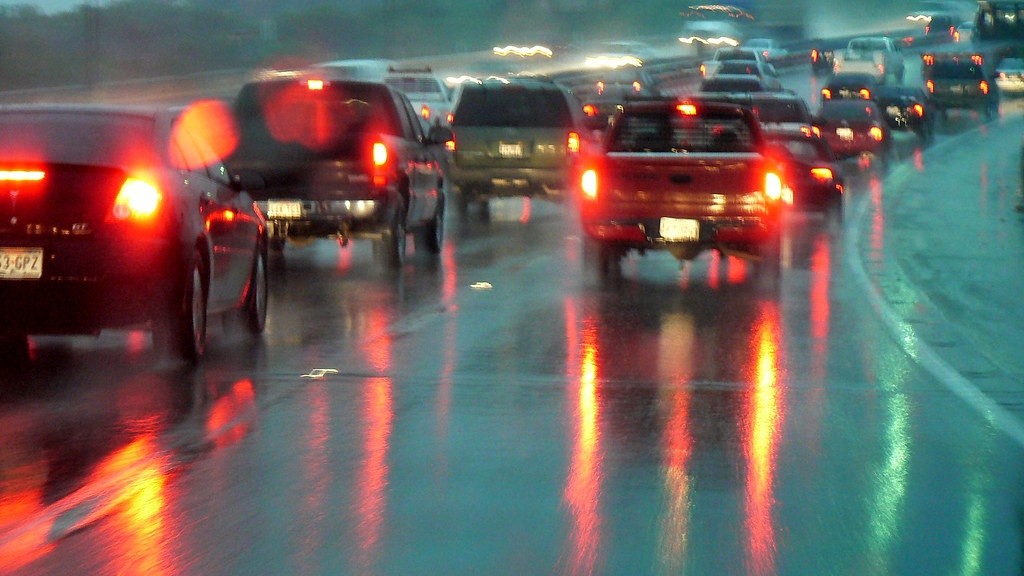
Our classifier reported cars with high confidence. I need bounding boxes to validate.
[0,99,272,365]
[290,1,1024,235]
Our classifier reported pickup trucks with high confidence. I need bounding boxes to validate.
[217,76,456,272]
[578,97,788,286]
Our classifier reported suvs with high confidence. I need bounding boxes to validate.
[440,78,595,223]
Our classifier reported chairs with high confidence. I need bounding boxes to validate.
[634,131,659,148]
[710,132,741,153]
